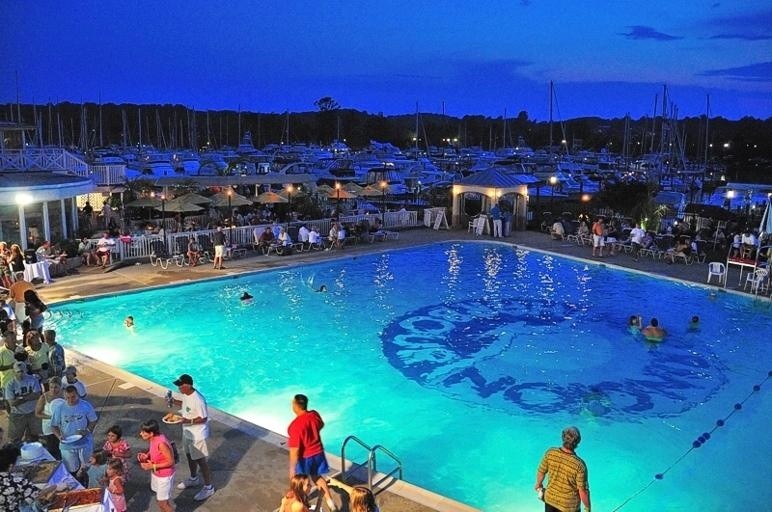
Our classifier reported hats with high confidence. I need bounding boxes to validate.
[174,374,193,386]
[14,361,25,372]
[63,365,77,374]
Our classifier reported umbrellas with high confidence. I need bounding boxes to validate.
[122,177,393,228]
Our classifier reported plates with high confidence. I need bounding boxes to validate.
[63,434,83,443]
[162,415,182,423]
[21,441,43,460]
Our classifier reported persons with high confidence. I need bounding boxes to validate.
[240,292,254,301]
[298,223,326,251]
[630,314,665,338]
[258,227,294,255]
[492,204,513,237]
[579,215,758,261]
[320,285,326,292]
[551,220,565,240]
[125,316,134,327]
[280,394,379,512]
[82,200,119,230]
[91,232,116,268]
[86,373,214,511]
[223,206,280,227]
[0,237,101,511]
[329,223,346,249]
[536,426,592,511]
[692,316,699,322]
[187,238,201,266]
[211,226,227,270]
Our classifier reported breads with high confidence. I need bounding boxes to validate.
[164,412,178,423]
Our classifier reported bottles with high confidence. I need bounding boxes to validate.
[167,390,174,408]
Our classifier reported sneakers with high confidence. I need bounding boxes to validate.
[177,476,200,489]
[194,487,214,501]
[330,505,340,511]
[218,266,226,270]
[212,266,217,268]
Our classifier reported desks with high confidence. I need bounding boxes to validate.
[22,258,53,287]
[17,438,116,512]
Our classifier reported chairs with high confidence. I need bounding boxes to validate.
[78,236,141,265]
[462,200,772,306]
[149,213,396,269]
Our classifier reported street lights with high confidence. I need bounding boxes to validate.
[159,193,166,255]
[334,182,341,224]
[417,180,422,222]
[286,186,293,235]
[381,181,386,228]
[227,190,234,247]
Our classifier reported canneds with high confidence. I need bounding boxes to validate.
[538,488,545,500]
